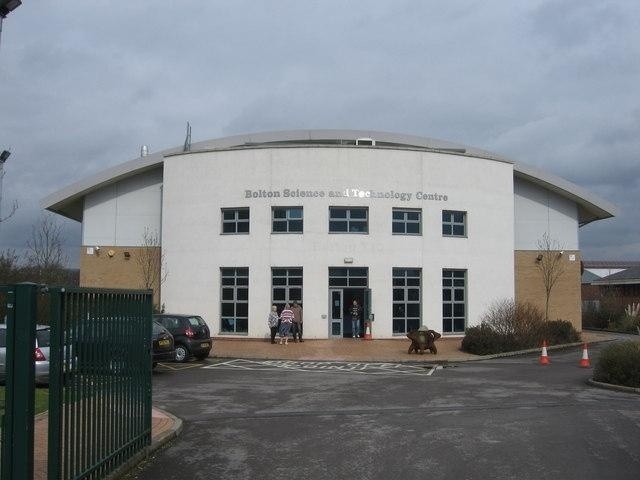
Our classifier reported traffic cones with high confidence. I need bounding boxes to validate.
[538,340,551,365]
[580,343,593,367]
[364,322,373,339]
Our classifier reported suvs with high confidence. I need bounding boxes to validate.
[1,312,212,386]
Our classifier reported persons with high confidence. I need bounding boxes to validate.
[348,299,364,339]
[290,299,306,343]
[278,301,294,345]
[267,305,281,345]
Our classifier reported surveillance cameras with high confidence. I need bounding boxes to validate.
[559,251,565,255]
[96,246,100,251]
